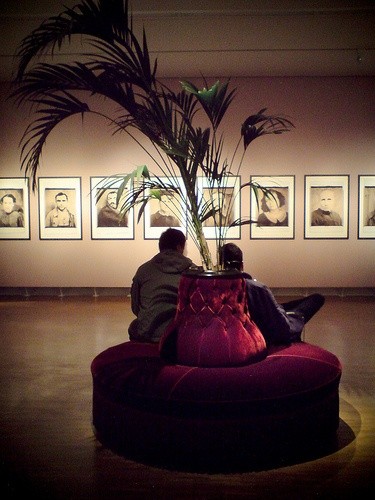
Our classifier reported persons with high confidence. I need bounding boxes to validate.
[128,228,197,344]
[256,188,288,226]
[150,191,181,227]
[45,191,76,228]
[367,214,375,225]
[0,194,24,227]
[311,190,342,226]
[204,191,232,227]
[219,243,325,346]
[98,191,128,227]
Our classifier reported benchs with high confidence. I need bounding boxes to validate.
[91,334,343,474]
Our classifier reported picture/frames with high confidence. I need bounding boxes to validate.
[38,176,82,241]
[357,174,375,240]
[0,177,31,240]
[89,176,135,241]
[303,174,350,240]
[196,176,241,241]
[143,176,190,241]
